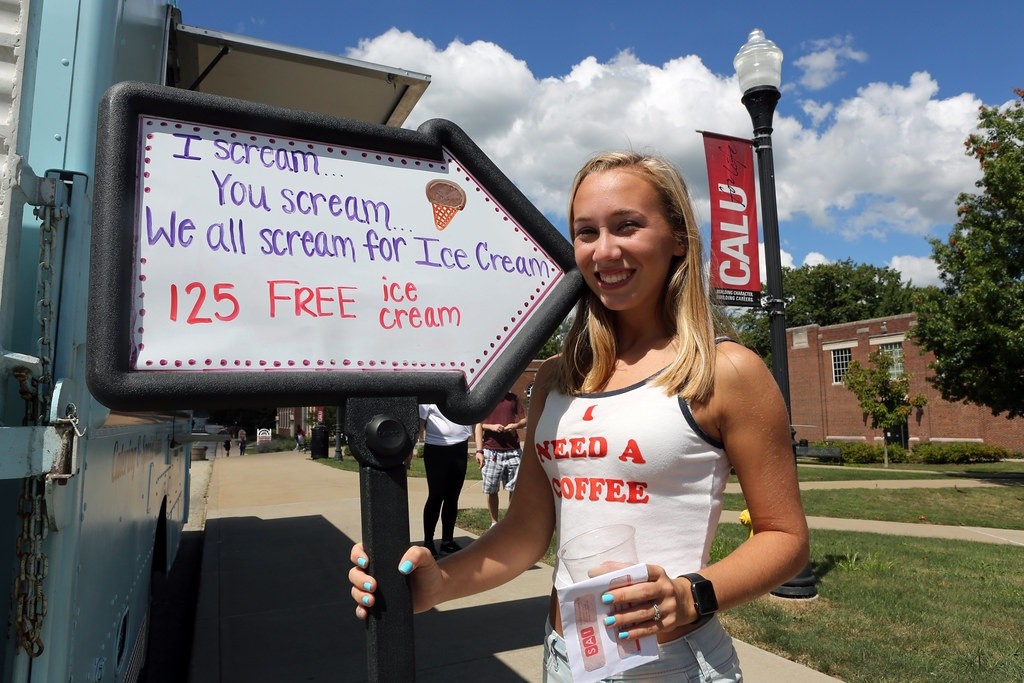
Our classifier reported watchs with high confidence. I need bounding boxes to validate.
[678,573,718,626]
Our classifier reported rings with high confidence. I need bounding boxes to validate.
[650,603,663,621]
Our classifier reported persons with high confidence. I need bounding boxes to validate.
[239,436,248,456]
[293,424,306,453]
[347,151,811,682]
[222,438,233,457]
[416,387,527,560]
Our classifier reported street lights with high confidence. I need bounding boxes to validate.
[733,26,819,601]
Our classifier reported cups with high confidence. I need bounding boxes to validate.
[557,524,635,584]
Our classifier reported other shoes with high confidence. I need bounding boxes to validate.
[439,538,462,555]
[424,542,438,559]
